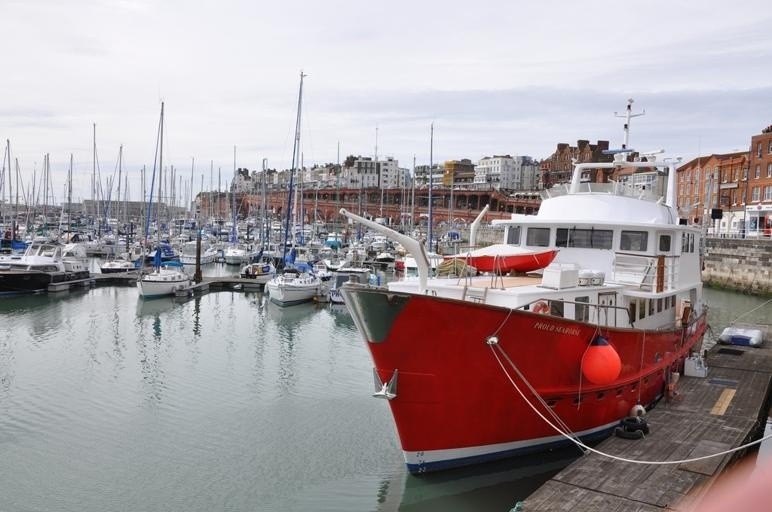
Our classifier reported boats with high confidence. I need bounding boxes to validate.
[330,302,356,329]
[263,297,328,347]
[372,451,590,508]
[334,94,719,477]
